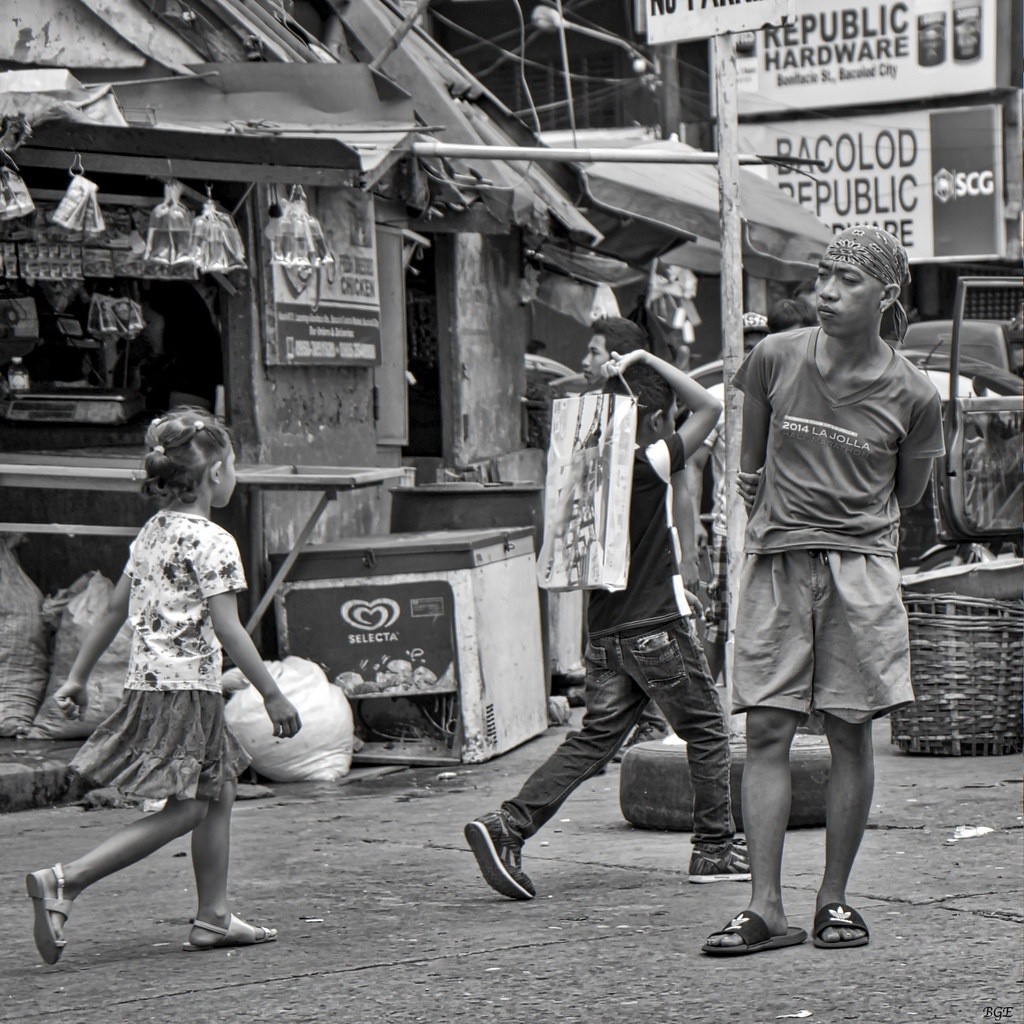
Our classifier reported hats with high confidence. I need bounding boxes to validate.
[743,311,772,334]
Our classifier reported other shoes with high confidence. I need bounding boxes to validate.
[611,723,668,762]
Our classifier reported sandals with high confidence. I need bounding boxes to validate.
[182,913,279,951]
[25,862,73,964]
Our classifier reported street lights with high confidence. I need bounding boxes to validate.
[529,5,681,138]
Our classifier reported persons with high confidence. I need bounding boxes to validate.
[527,340,546,356]
[464,349,752,899]
[768,297,818,335]
[702,226,947,955]
[26,404,301,964]
[138,289,224,411]
[683,311,768,682]
[582,316,700,761]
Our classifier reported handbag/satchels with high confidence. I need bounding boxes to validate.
[534,365,639,593]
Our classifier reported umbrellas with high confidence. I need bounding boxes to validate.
[574,139,833,309]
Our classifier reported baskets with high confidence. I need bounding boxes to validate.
[888,590,1024,757]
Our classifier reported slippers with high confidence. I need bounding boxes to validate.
[701,910,808,955]
[810,902,870,949]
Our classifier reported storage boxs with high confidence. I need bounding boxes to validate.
[541,393,639,592]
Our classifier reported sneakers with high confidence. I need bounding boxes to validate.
[687,840,752,883]
[463,811,536,898]
